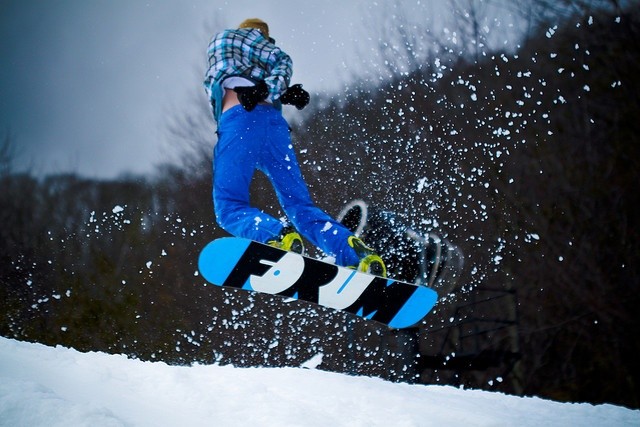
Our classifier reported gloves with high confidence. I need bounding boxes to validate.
[233,83,269,112]
[280,84,310,110]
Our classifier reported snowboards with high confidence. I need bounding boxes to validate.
[199,238,438,329]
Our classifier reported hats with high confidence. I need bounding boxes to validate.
[238,18,269,39]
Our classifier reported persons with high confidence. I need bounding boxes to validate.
[202,17,387,277]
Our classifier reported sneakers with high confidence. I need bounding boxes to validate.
[268,226,305,255]
[346,254,387,280]
